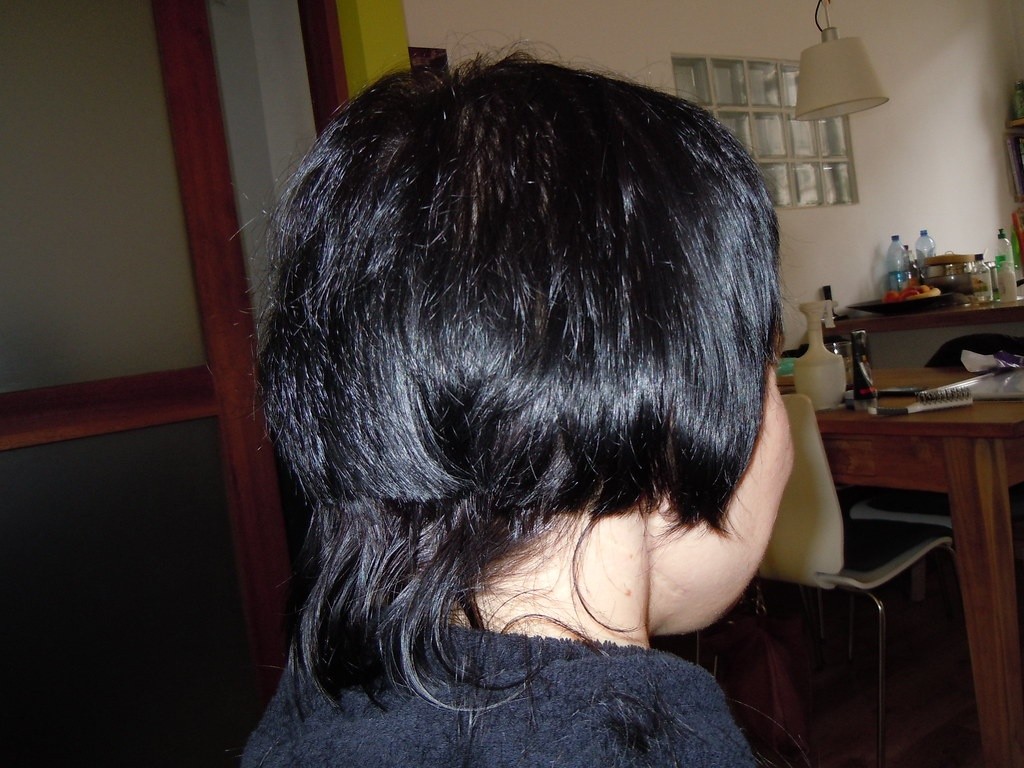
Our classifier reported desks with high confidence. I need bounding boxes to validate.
[772,365,1024,768]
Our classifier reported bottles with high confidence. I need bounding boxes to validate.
[971,253,994,303]
[994,228,1015,284]
[914,230,934,278]
[886,235,911,294]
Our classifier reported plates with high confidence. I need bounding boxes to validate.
[846,292,952,315]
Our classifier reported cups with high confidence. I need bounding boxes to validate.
[823,340,854,387]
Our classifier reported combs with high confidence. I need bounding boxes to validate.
[867,387,973,414]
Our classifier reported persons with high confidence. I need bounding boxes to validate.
[239,55,794,768]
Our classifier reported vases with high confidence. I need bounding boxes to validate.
[794,301,846,410]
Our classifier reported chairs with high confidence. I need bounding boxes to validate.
[707,333,1024,767]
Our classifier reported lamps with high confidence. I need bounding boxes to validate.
[795,0,889,120]
